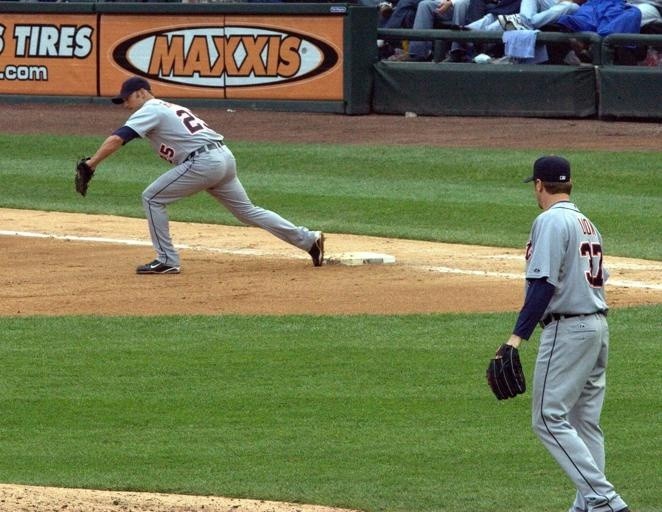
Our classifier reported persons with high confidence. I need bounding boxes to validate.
[75,77,326,274]
[486,156,630,512]
[376,0,662,66]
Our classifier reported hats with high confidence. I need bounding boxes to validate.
[110,75,150,105]
[521,154,573,185]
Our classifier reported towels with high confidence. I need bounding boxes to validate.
[503,29,549,65]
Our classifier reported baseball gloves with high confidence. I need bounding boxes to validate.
[75,157,95,195]
[487,345,526,400]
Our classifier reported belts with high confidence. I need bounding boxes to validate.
[539,311,603,329]
[183,141,224,163]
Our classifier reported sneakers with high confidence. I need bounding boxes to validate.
[308,231,325,266]
[136,258,180,275]
